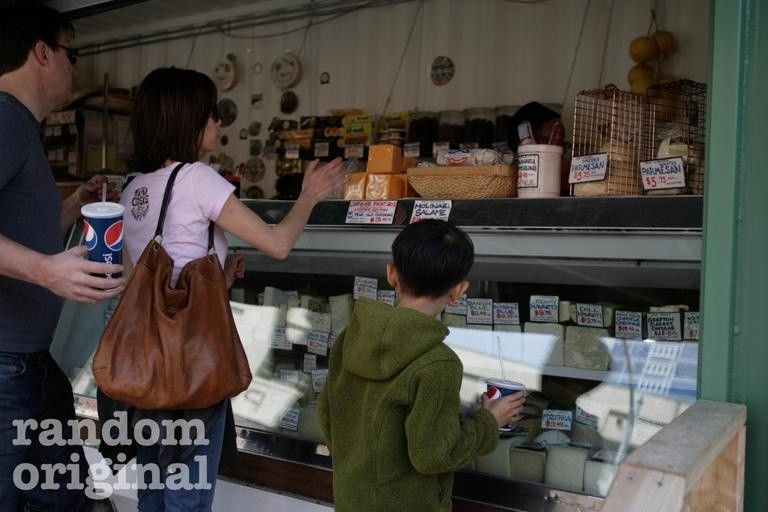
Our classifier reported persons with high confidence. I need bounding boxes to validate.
[0,2,126,512]
[316,218,526,511]
[95,64,353,512]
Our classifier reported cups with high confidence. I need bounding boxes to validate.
[80,201,125,294]
[483,375,525,432]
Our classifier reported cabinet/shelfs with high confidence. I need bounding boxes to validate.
[46,198,706,512]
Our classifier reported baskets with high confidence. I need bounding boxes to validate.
[406,165,516,199]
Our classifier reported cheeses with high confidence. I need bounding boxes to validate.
[231,286,699,500]
[342,144,415,200]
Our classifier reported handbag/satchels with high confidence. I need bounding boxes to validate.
[93,238,252,412]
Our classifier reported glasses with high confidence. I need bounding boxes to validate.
[51,43,79,64]
[211,105,221,121]
